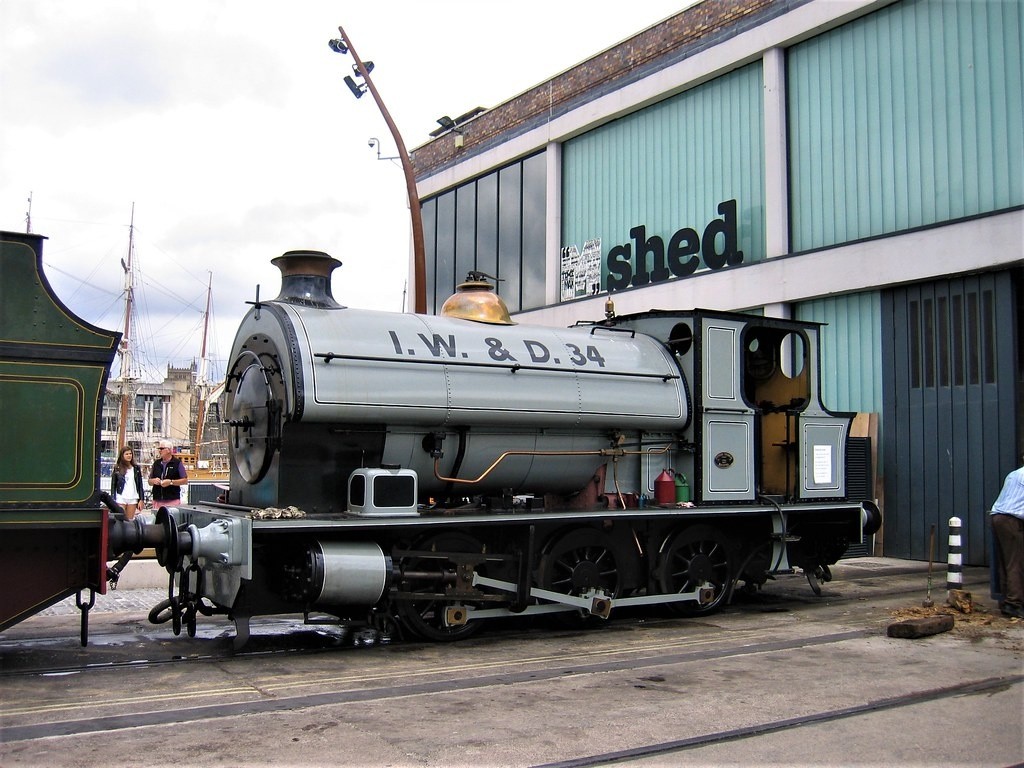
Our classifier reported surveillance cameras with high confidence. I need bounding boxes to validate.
[368,140,375,147]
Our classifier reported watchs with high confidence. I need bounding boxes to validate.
[169,479,173,485]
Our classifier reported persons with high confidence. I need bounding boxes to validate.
[110,446,144,520]
[148,440,188,510]
[989,467,1024,616]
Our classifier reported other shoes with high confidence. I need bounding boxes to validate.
[1001,603,1024,618]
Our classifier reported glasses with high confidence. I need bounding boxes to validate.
[158,447,167,450]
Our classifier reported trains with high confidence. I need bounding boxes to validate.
[0,219,883,649]
